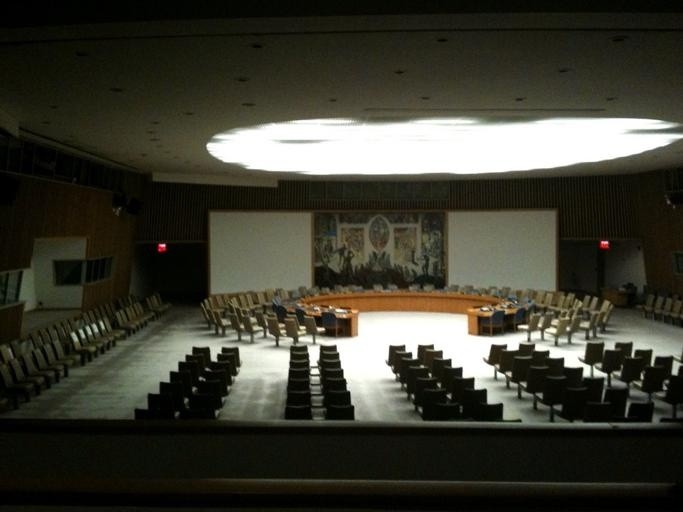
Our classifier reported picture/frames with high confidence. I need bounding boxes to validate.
[310,209,450,289]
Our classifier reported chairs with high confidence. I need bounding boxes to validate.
[636,294,683,328]
[132,344,240,419]
[0,291,172,416]
[200,279,364,346]
[385,342,683,424]
[372,281,616,347]
[282,343,356,420]
